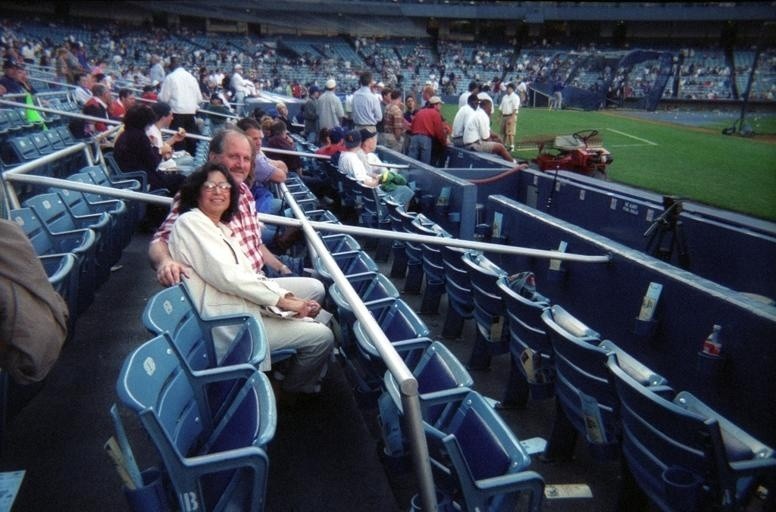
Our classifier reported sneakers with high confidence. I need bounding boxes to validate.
[508,145,515,152]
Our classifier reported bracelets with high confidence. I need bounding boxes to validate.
[277,263,288,276]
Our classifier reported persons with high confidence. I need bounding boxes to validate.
[147,127,348,354]
[518,35,775,114]
[166,164,340,418]
[2,213,72,470]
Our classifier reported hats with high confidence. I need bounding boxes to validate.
[360,128,377,140]
[331,128,347,143]
[235,64,243,69]
[309,86,320,94]
[504,83,516,91]
[325,79,336,89]
[2,61,27,71]
[429,96,445,105]
[344,131,362,148]
[64,35,84,51]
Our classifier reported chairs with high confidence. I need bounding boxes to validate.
[1,35,775,110]
[1,164,774,511]
[1,106,517,177]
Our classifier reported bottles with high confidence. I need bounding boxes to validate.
[702,324,722,356]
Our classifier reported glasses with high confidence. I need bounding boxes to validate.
[202,180,232,193]
